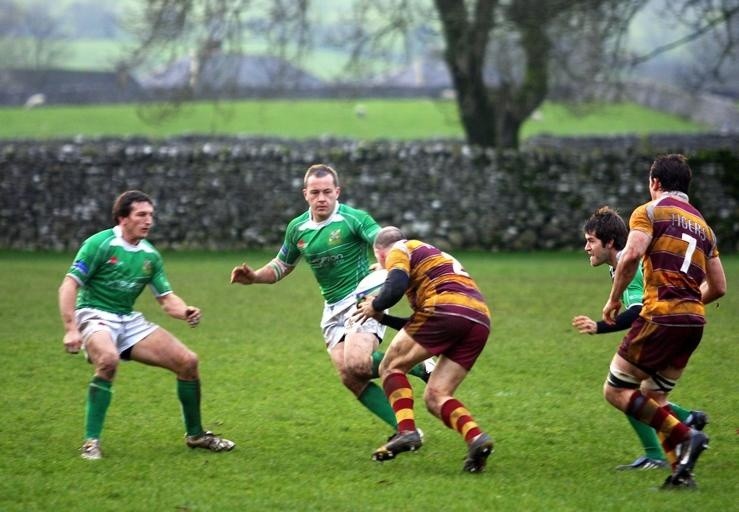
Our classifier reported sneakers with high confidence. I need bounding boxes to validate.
[421,355,436,384]
[78,437,102,461]
[613,455,667,473]
[673,411,711,483]
[371,427,425,464]
[184,429,236,453]
[647,474,698,494]
[461,433,495,475]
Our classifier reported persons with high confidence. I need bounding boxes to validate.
[351,226,494,473]
[231,165,437,438]
[58,189,236,459]
[601,155,727,490]
[571,206,709,470]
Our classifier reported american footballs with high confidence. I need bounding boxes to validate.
[354,268,388,303]
